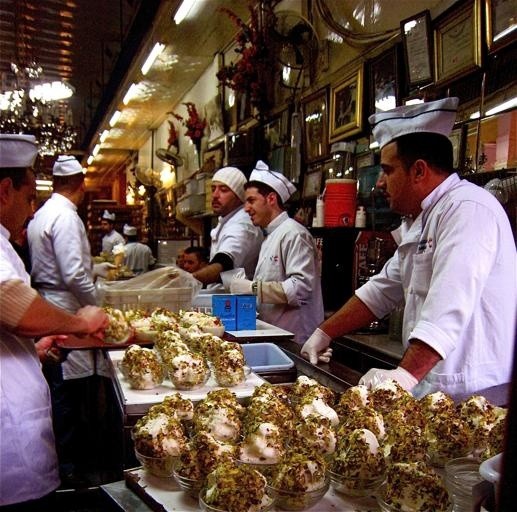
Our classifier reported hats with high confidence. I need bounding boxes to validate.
[53,155,83,176]
[211,166,247,203]
[123,224,137,236]
[249,160,297,203]
[0,134,38,169]
[103,210,115,221]
[368,97,458,149]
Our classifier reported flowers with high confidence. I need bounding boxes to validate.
[166,102,207,151]
[215,4,282,112]
[167,120,180,154]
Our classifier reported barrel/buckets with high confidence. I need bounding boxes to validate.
[357,263,388,335]
[322,178,358,227]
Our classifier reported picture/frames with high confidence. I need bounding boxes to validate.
[364,42,402,131]
[302,168,322,199]
[353,149,376,183]
[431,0,482,89]
[448,122,468,174]
[485,0,517,55]
[328,61,364,145]
[299,83,334,169]
[247,103,301,185]
[400,9,432,92]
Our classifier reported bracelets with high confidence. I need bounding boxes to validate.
[252,281,258,297]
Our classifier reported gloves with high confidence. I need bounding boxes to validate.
[360,367,417,398]
[230,278,254,296]
[301,328,332,366]
[93,262,121,278]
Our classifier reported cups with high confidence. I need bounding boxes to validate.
[444,456,483,512]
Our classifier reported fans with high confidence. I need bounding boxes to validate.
[155,147,189,170]
[265,10,329,74]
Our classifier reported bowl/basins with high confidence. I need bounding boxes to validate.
[211,367,252,387]
[167,368,211,391]
[117,361,130,379]
[202,326,225,337]
[262,466,330,511]
[128,368,163,389]
[325,460,387,497]
[172,460,209,499]
[198,487,278,512]
[236,459,279,476]
[134,446,180,479]
[376,483,454,512]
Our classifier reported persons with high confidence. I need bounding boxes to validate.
[120,224,156,274]
[0,133,110,510]
[300,96,516,407]
[183,247,210,273]
[177,247,187,269]
[102,210,126,263]
[230,160,324,346]
[191,166,265,286]
[27,155,124,490]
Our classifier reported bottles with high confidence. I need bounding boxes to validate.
[355,206,366,227]
[316,196,324,227]
